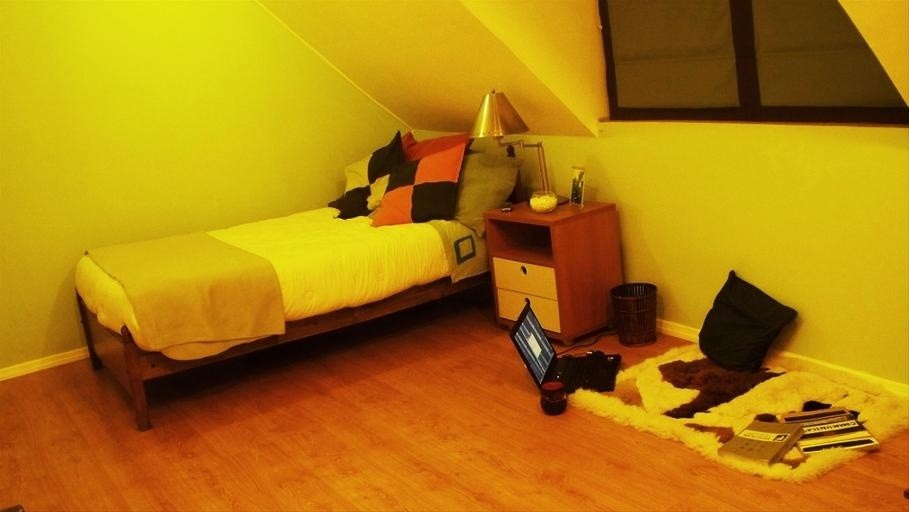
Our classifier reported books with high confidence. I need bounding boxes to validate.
[717,418,805,467]
[780,406,882,458]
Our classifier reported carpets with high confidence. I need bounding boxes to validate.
[568,344,909,486]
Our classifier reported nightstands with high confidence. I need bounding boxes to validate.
[483,194,623,345]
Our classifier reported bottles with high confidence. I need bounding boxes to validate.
[539,380,567,416]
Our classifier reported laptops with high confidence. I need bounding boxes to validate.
[510,303,618,393]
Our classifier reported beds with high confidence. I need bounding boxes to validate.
[74,133,490,432]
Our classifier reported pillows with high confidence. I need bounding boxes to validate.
[328,128,521,237]
[697,272,798,372]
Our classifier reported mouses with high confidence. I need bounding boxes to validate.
[587,350,604,356]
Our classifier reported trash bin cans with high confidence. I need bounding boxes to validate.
[610,283,657,347]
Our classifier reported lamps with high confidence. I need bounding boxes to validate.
[469,88,569,206]
